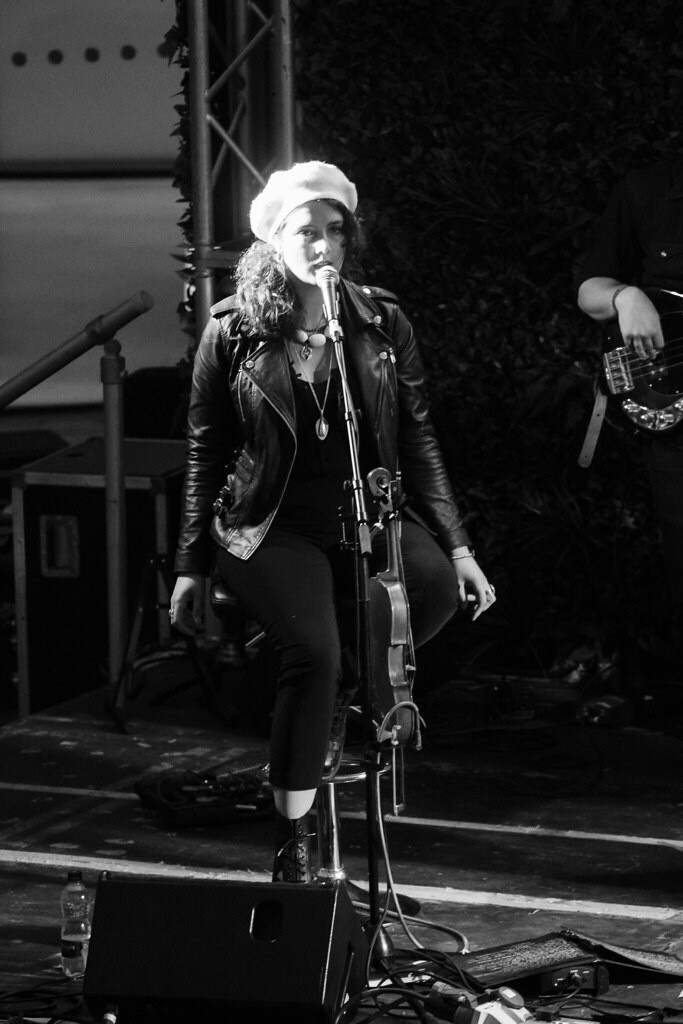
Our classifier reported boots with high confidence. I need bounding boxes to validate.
[271,810,316,885]
[320,672,360,781]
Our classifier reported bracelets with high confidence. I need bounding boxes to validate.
[612,285,631,311]
[451,549,475,559]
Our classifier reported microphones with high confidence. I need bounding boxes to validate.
[315,265,341,342]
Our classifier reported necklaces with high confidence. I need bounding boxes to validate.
[289,312,336,440]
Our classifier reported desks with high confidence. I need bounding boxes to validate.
[12,436,189,721]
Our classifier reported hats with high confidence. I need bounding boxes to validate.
[250,160,358,244]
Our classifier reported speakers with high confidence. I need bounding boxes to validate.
[83,871,371,1024]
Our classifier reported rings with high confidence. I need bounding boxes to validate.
[490,585,495,594]
[169,610,173,618]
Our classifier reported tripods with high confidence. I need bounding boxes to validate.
[333,340,451,1024]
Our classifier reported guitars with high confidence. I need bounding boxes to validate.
[597,274,683,437]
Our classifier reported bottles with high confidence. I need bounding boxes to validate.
[60,867,91,978]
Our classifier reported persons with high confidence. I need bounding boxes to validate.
[168,162,501,883]
[569,153,683,664]
